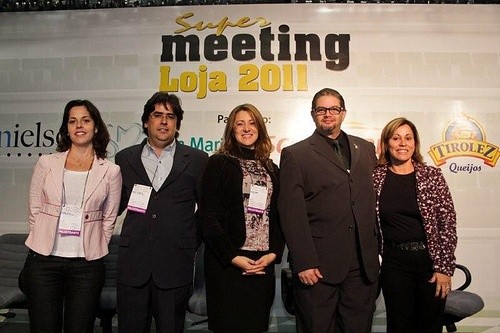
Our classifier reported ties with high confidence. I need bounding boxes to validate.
[334,140,346,168]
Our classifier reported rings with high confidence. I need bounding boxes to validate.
[445,287,450,290]
[304,281,308,284]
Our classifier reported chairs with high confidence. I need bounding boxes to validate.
[0,234,292,333]
[443,264,484,333]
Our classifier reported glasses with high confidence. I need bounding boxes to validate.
[149,114,179,120]
[312,106,346,115]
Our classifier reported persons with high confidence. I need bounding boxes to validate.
[277,87,383,333]
[18,100,122,332]
[200,102,284,333]
[114,90,209,333]
[371,117,458,333]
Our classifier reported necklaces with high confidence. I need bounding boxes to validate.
[68,152,94,167]
